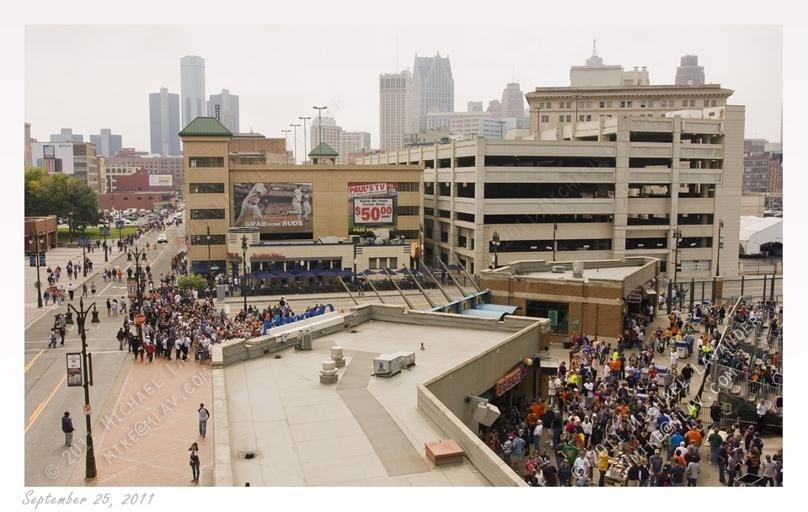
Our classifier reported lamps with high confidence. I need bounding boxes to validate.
[465,395,502,427]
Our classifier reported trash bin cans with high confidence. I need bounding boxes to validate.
[297,330,313,350]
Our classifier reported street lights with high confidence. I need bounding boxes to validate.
[492,230,500,267]
[673,228,682,282]
[65,296,100,476]
[282,106,326,164]
[29,229,46,306]
[126,245,149,313]
[241,234,249,310]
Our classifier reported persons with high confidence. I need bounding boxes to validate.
[60,411,77,447]
[187,442,199,484]
[233,183,312,227]
[43,208,327,366]
[478,287,783,486]
[358,280,364,295]
[197,402,211,437]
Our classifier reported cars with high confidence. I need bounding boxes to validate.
[158,233,168,244]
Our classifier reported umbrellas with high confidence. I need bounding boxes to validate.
[319,265,462,278]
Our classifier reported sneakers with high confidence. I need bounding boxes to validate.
[191,479,199,484]
